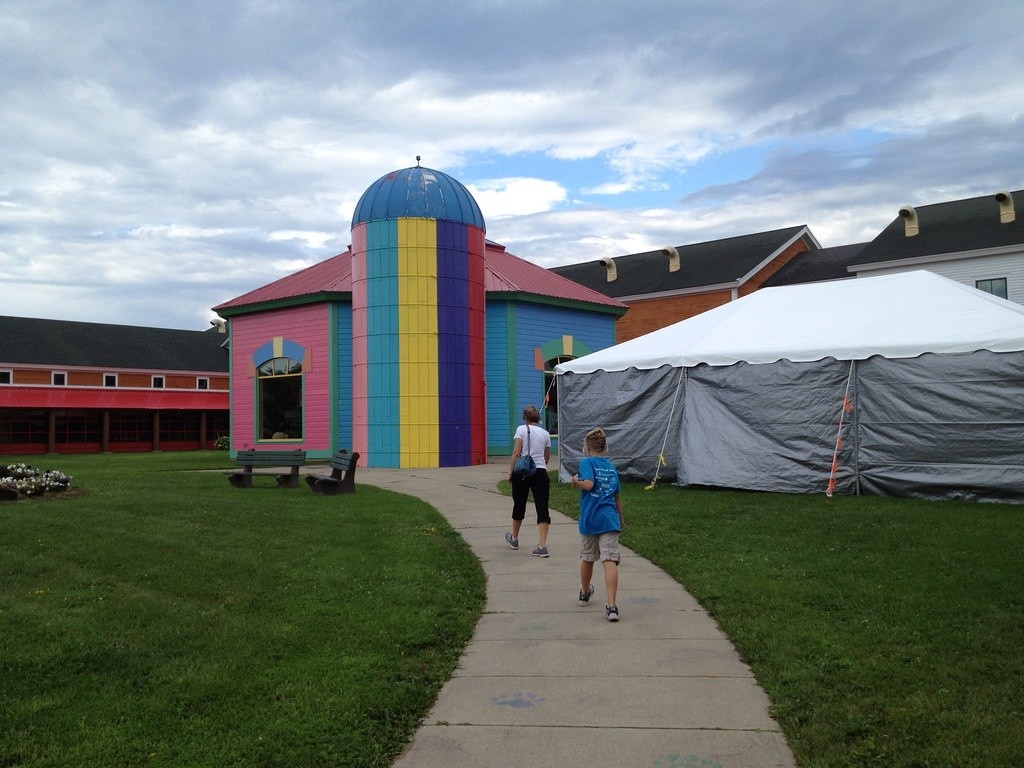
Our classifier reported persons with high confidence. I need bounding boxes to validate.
[571,427,625,621]
[505,405,551,558]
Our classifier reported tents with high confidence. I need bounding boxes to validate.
[555,268,1024,502]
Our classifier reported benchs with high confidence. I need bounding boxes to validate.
[224,448,307,488]
[305,449,360,494]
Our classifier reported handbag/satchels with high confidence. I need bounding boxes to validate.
[513,424,537,479]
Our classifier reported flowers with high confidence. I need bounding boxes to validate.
[0,462,74,497]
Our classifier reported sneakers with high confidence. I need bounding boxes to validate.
[577,583,594,606]
[531,544,549,557]
[605,604,620,620]
[504,531,519,549]
